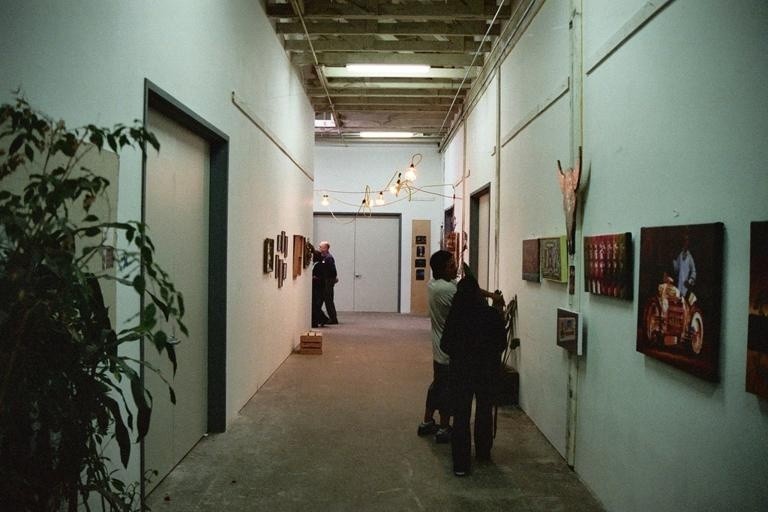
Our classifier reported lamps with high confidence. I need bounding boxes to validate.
[321,154,417,207]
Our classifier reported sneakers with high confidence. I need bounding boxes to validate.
[313,317,338,327]
[418,420,452,442]
[455,464,467,475]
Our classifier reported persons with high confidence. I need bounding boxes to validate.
[311,250,330,328]
[668,232,695,295]
[440,275,505,476]
[417,249,506,443]
[317,240,341,325]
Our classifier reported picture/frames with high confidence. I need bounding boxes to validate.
[263,231,288,288]
